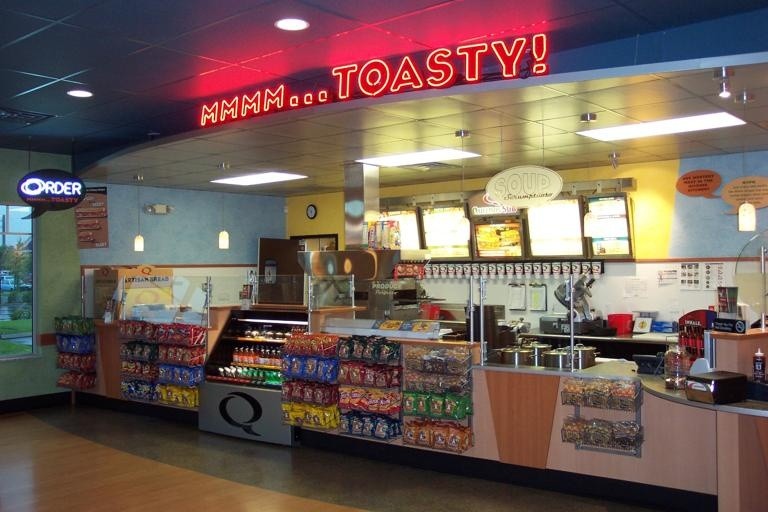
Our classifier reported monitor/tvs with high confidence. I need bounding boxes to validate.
[470,213,526,259]
[524,195,587,259]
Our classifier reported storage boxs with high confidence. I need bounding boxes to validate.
[94,264,173,319]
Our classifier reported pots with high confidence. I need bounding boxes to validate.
[491,340,602,369]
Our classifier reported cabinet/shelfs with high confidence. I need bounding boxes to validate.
[59,313,96,392]
[115,320,158,404]
[281,331,337,434]
[159,322,205,411]
[340,334,403,440]
[557,373,644,459]
[403,344,476,454]
[205,309,321,387]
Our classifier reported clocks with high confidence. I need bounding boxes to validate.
[307,205,317,219]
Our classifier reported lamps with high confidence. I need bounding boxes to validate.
[455,130,471,241]
[580,113,599,237]
[735,91,757,232]
[133,176,144,252]
[608,152,620,169]
[146,204,174,215]
[712,67,735,99]
[217,161,230,249]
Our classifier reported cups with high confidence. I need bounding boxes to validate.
[423,261,601,278]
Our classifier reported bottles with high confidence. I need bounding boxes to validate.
[663,344,691,389]
[752,348,766,385]
[230,345,284,367]
